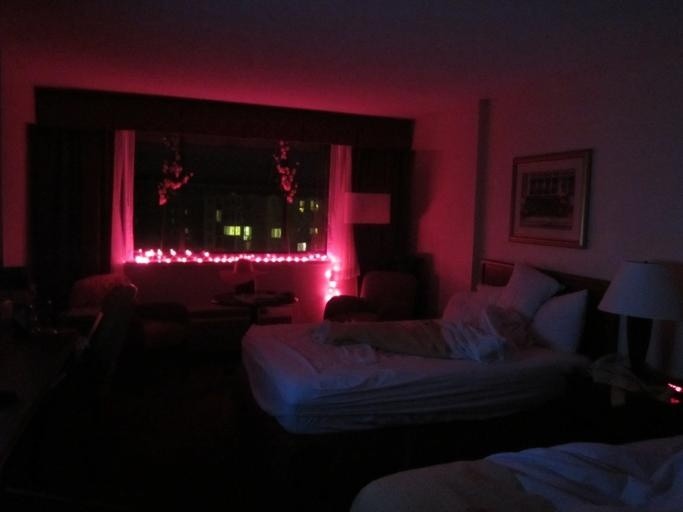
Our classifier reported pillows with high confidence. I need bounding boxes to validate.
[495,263,561,320]
[475,283,507,305]
[530,289,590,353]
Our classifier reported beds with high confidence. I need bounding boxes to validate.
[240,261,620,435]
[349,436,683,511]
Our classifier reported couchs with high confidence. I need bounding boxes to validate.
[323,269,418,320]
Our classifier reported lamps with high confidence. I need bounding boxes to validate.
[344,190,391,277]
[598,261,683,378]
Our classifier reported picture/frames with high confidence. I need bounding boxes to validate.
[510,148,593,249]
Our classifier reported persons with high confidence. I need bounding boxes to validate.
[156,161,195,248]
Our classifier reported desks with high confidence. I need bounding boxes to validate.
[215,289,292,323]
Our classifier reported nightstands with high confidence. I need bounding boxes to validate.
[566,361,682,440]
